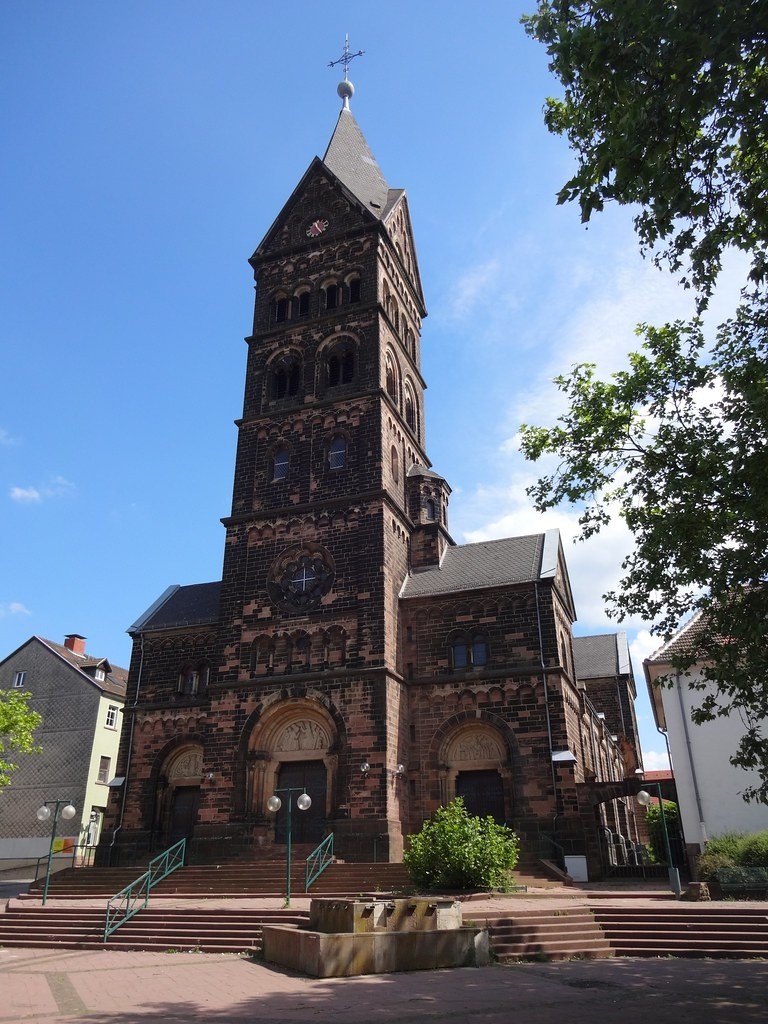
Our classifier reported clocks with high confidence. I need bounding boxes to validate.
[305,218,329,238]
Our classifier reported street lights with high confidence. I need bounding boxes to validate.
[267,788,312,906]
[638,783,676,891]
[36,799,76,904]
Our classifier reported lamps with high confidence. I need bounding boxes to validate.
[205,772,216,786]
[392,763,405,780]
[360,762,370,779]
